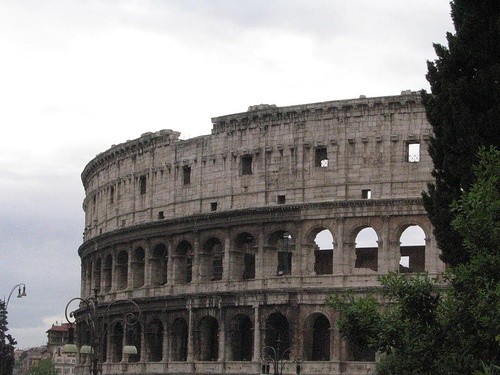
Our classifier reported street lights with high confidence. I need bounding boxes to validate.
[3,283,26,374]
